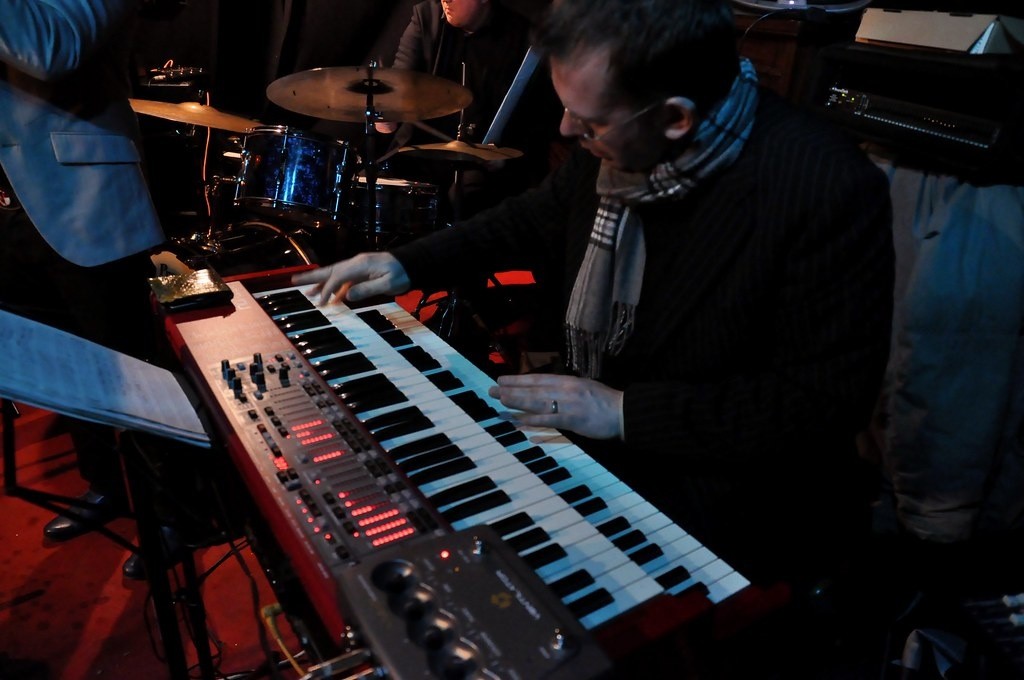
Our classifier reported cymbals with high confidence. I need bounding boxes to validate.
[126,99,267,135]
[265,62,474,122]
[395,140,524,164]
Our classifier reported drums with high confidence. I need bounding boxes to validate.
[171,126,445,267]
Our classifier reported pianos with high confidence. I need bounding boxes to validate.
[152,261,789,680]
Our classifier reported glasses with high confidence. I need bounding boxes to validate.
[565,93,671,146]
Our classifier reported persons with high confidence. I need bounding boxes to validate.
[379,0,544,201]
[0,0,184,579]
[290,0,894,585]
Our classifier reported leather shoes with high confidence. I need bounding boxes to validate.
[43,489,116,541]
[124,547,154,584]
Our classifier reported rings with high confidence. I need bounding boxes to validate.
[552,400,557,414]
